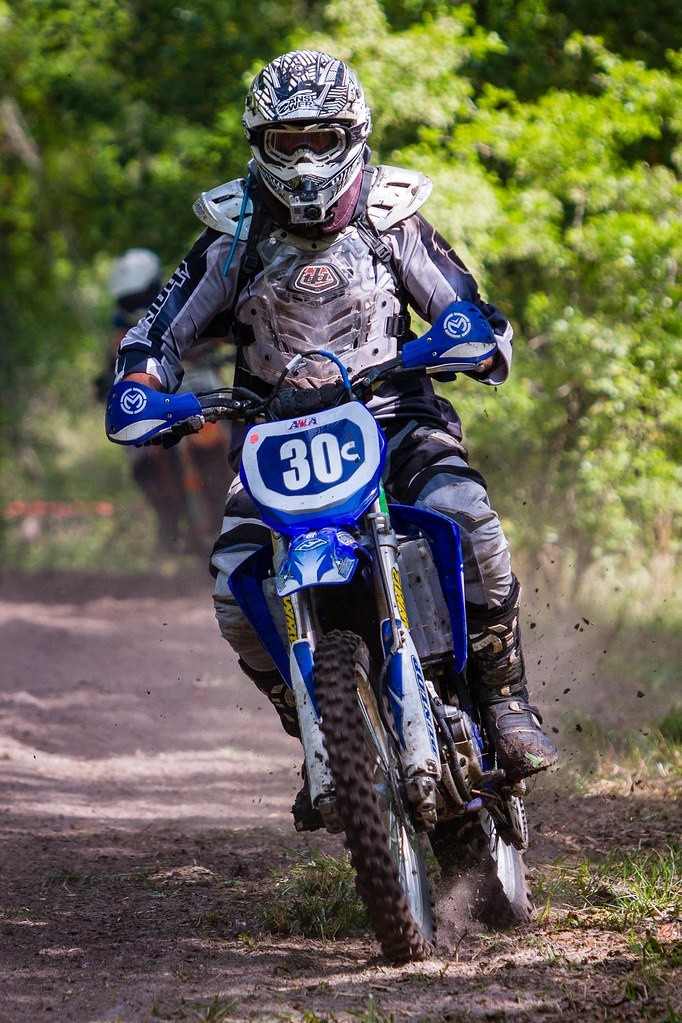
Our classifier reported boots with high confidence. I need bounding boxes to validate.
[465,571,559,780]
[238,657,321,822]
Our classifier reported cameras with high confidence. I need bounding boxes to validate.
[289,193,325,223]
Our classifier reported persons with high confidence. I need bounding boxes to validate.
[103,250,235,555]
[112,48,559,834]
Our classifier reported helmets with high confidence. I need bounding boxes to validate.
[241,49,372,224]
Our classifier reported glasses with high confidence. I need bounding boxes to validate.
[261,126,348,167]
